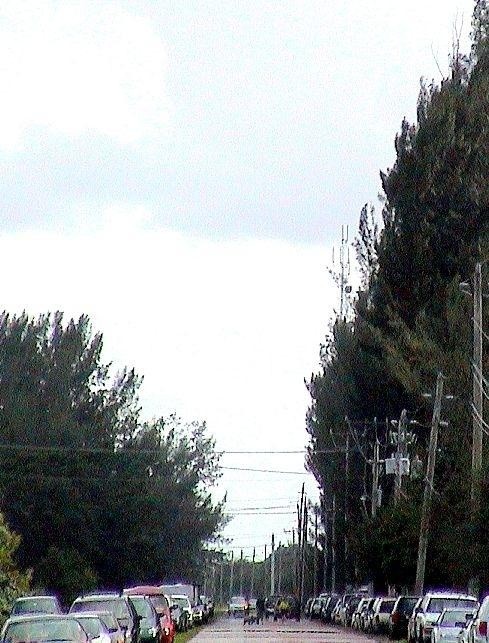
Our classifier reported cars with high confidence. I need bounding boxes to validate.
[0,581,215,643]
[228,593,302,624]
[304,588,489,643]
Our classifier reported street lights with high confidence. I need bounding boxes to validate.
[413,372,455,596]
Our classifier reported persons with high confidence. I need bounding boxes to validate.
[242,589,302,625]
